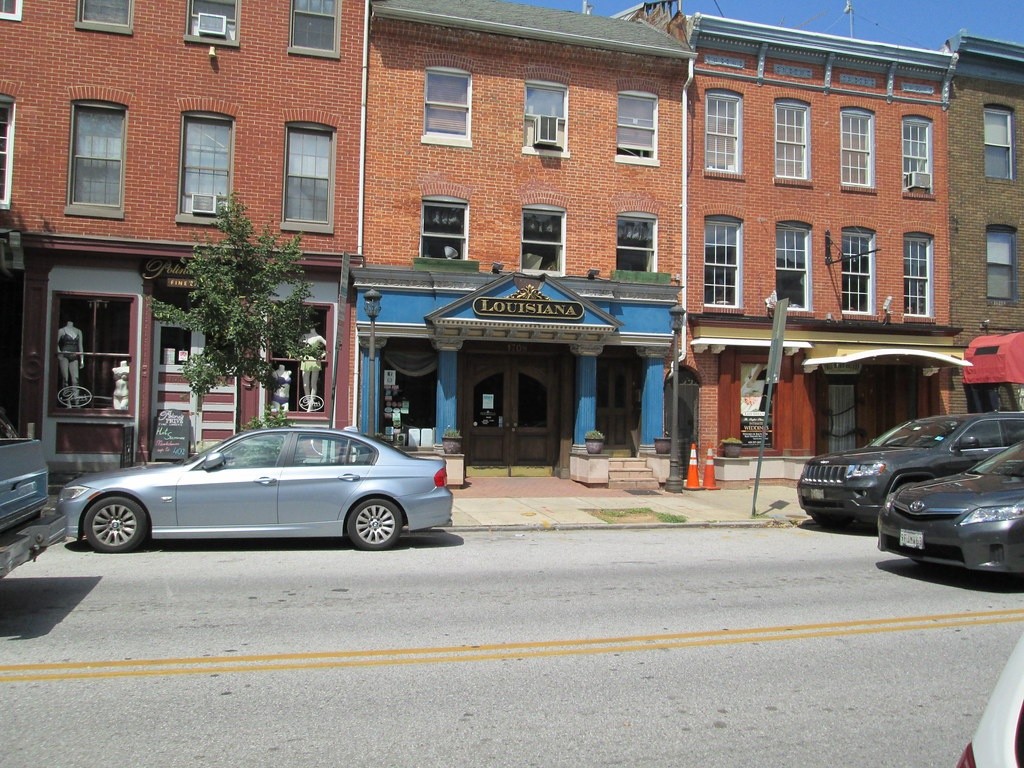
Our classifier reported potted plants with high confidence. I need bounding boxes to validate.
[441,424,463,454]
[584,431,605,454]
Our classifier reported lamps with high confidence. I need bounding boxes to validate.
[208,46,216,58]
[492,260,504,273]
[588,268,600,277]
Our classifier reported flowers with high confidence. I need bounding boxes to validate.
[723,436,742,444]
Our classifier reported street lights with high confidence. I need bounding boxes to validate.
[667,306,689,490]
[363,287,383,439]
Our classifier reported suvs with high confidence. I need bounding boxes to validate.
[796,410,1023,533]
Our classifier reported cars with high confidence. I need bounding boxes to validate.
[877,438,1024,572]
[959,631,1024,768]
[57,426,453,551]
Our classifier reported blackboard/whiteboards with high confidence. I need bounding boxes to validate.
[151,409,191,461]
[123,427,134,468]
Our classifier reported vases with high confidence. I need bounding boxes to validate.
[724,443,740,457]
[653,437,671,453]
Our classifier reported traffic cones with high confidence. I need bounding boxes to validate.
[684,443,701,491]
[702,442,720,491]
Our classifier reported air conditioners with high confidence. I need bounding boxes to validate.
[198,12,227,37]
[191,192,223,216]
[907,169,931,189]
[533,115,557,143]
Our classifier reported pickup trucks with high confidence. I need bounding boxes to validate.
[0,438,68,582]
